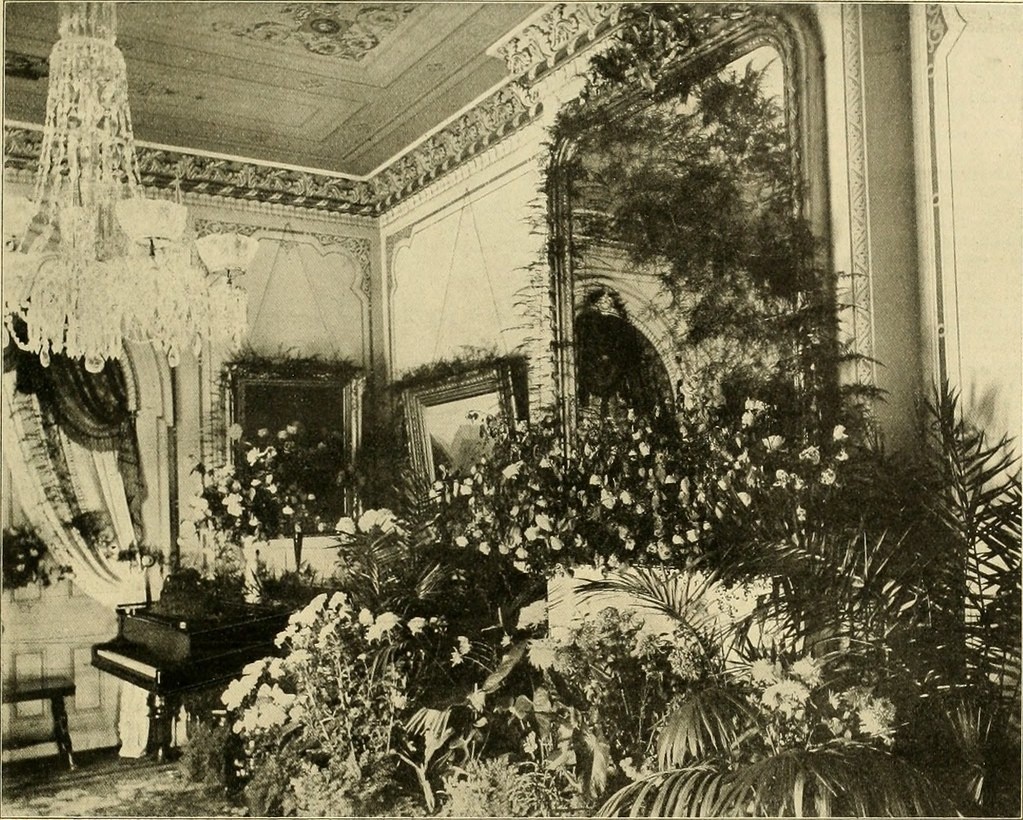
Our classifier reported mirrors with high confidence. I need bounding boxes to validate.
[551,3,837,459]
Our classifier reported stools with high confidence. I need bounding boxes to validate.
[2,676,80,772]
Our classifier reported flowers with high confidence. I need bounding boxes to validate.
[165,373,1021,818]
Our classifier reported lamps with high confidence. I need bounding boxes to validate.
[3,2,261,374]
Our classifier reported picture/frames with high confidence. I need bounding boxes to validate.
[401,363,520,489]
[230,368,367,534]
